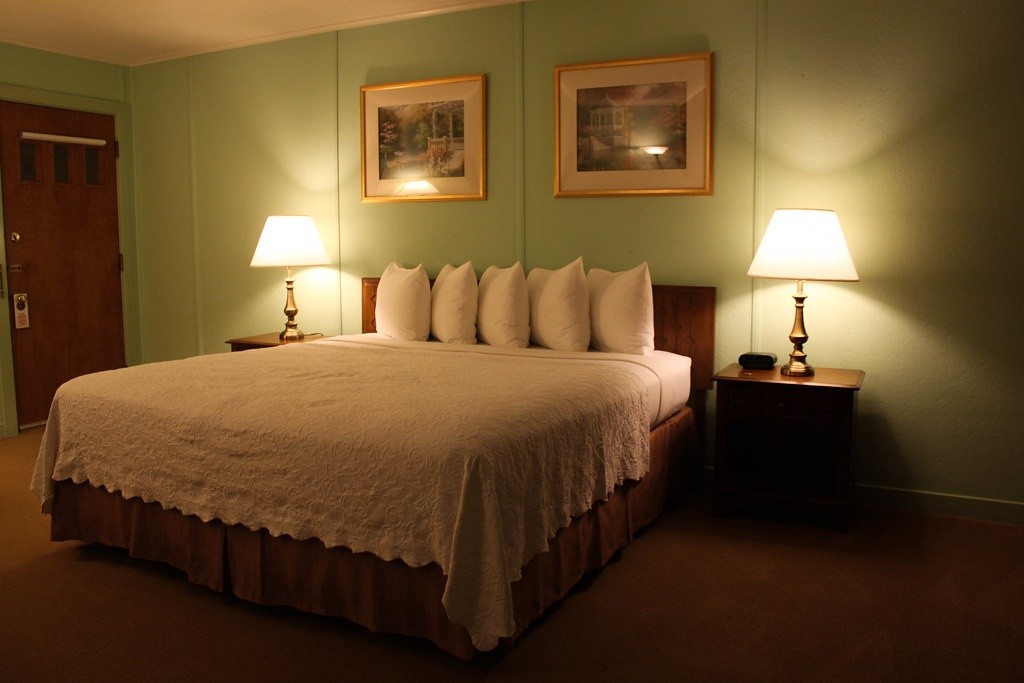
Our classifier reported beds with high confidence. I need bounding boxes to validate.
[30,255,717,665]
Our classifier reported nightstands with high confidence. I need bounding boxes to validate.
[709,364,865,526]
[225,332,336,353]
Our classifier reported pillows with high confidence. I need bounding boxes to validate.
[528,256,590,352]
[478,261,530,348]
[431,260,477,345]
[374,261,431,342]
[587,261,654,356]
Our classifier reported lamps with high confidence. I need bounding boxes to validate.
[746,208,859,376]
[250,215,332,340]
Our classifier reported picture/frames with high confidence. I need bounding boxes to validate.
[554,52,715,200]
[359,72,488,203]
